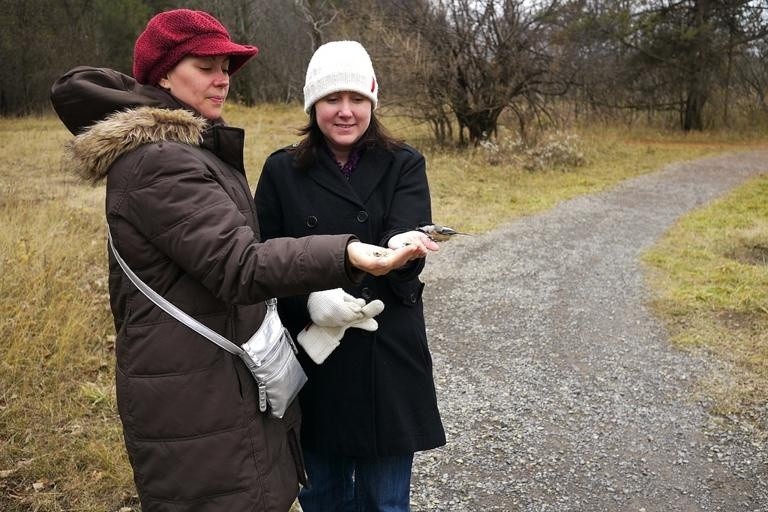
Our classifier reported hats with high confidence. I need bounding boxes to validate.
[133,9,257,86]
[303,41,377,112]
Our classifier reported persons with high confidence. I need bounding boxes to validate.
[49,9,420,512]
[258,40,445,511]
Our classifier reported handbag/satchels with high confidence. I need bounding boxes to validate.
[240,298,310,420]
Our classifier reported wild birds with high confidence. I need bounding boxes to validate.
[415,224,471,242]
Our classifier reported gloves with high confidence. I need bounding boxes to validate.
[296,289,385,365]
